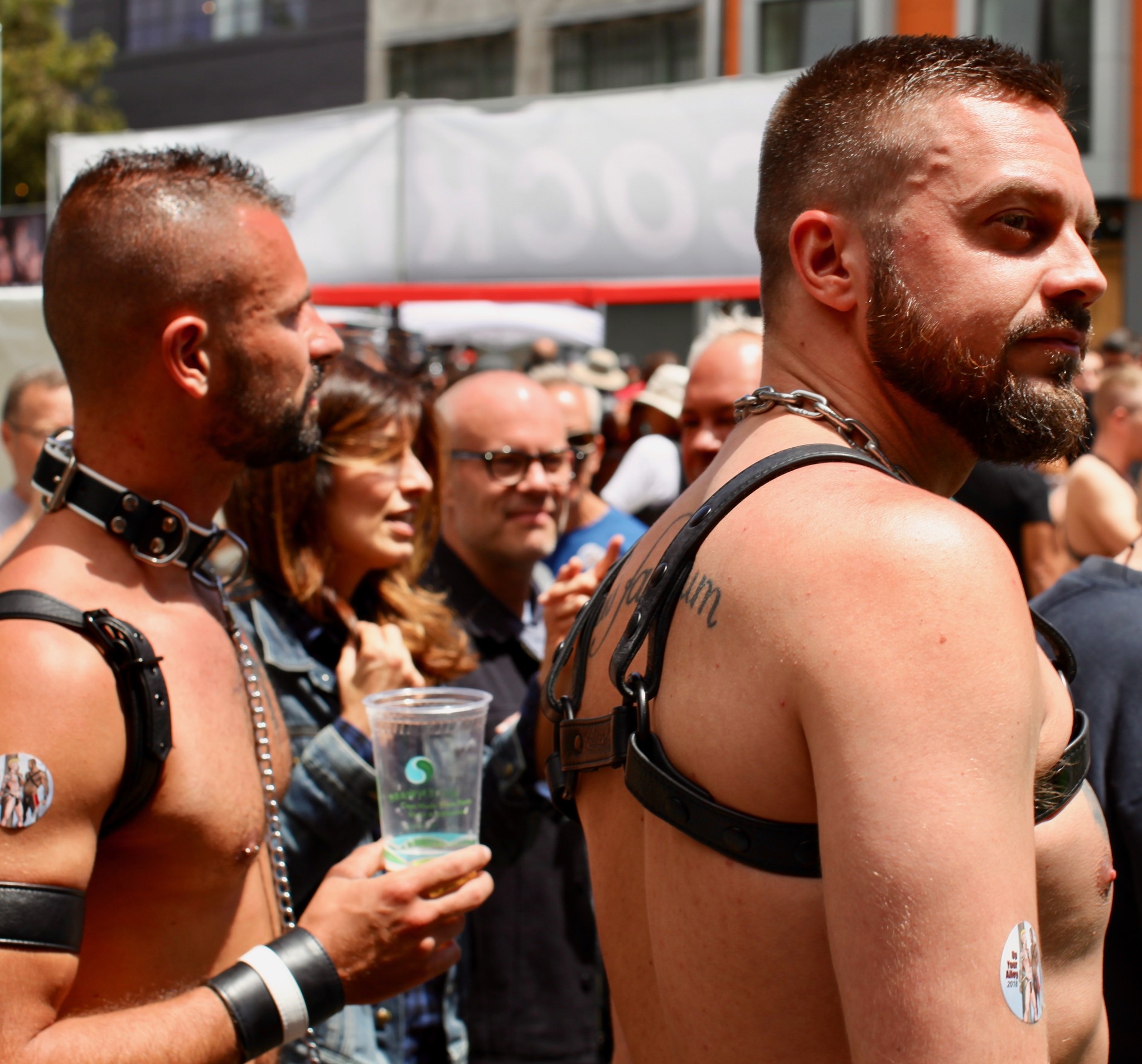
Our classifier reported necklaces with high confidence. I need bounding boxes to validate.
[735,385,917,482]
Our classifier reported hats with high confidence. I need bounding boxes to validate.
[635,362,693,419]
[569,345,632,389]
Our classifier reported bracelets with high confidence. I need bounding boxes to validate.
[199,927,347,1063]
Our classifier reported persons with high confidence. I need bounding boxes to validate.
[22,760,49,823]
[0,35,1142,1064]
[1019,930,1038,1023]
[0,757,24,828]
[1028,928,1042,1014]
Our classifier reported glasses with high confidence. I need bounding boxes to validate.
[452,444,579,486]
[567,432,605,473]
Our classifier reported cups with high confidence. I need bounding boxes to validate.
[362,687,494,872]
